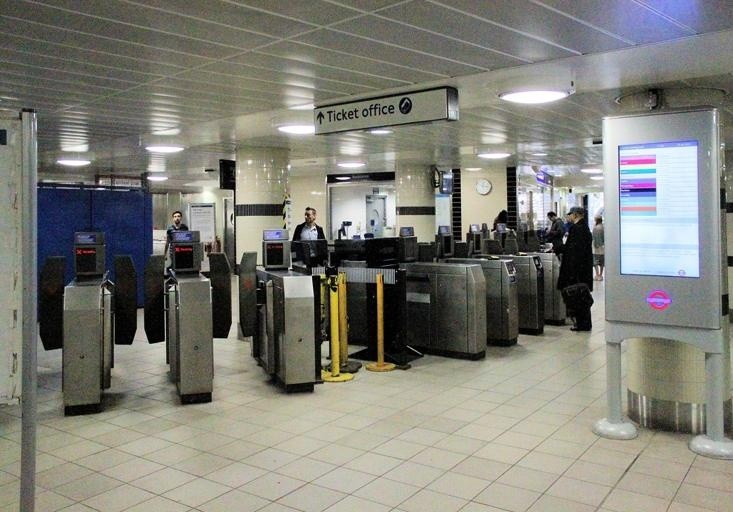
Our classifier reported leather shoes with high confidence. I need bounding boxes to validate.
[570,324,592,331]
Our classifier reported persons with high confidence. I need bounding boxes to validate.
[561,213,574,246]
[163,210,189,266]
[491,210,511,248]
[543,211,563,257]
[291,207,329,269]
[555,206,593,332]
[591,214,604,281]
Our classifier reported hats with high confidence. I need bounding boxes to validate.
[567,207,584,215]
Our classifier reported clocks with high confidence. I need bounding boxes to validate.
[476,178,492,196]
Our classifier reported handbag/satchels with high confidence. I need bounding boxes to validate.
[561,282,594,322]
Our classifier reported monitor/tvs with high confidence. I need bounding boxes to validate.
[219,159,236,190]
[439,171,454,194]
